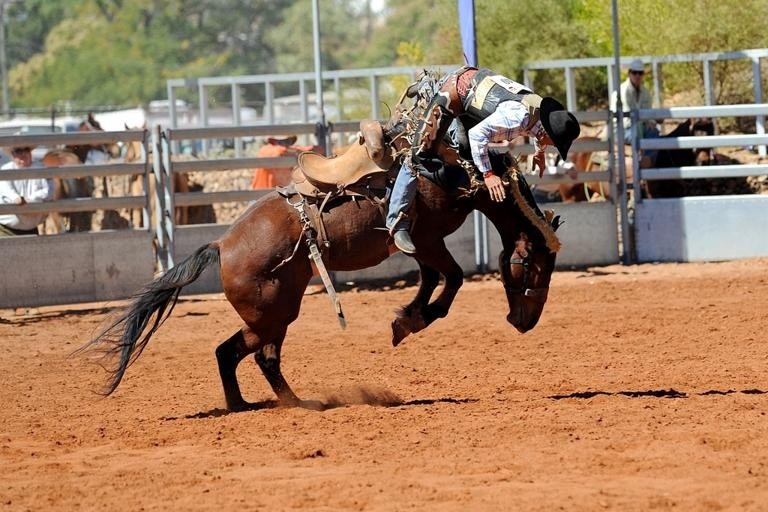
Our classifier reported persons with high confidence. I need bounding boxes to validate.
[386,64,582,254]
[609,59,664,167]
[0,130,56,237]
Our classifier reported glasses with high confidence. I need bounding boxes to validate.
[629,70,645,76]
[13,146,30,154]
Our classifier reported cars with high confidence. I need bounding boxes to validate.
[0,116,80,160]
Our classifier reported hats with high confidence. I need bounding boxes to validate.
[539,96,581,161]
[267,134,297,145]
[628,58,646,71]
[1,132,39,156]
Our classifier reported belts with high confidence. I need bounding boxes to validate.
[449,67,469,112]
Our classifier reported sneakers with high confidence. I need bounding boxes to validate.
[386,227,417,254]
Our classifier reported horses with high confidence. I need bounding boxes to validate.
[67,139,565,414]
[566,116,719,201]
[37,124,192,232]
